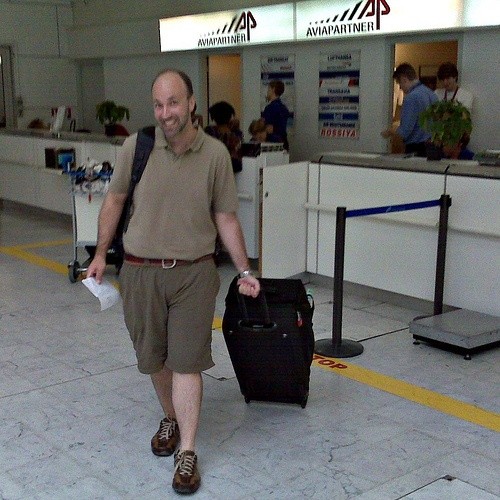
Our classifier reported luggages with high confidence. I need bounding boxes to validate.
[222,273,315,408]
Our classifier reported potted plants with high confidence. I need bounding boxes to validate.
[420,101,472,160]
[94,100,130,136]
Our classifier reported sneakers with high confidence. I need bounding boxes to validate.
[172,449,201,494]
[151,413,179,456]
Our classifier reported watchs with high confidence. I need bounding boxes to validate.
[240,269,252,277]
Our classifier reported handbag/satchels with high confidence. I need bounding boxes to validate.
[112,196,135,270]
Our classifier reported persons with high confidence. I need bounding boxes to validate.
[231,119,243,140]
[442,129,474,161]
[250,79,289,152]
[87,70,261,495]
[203,101,242,159]
[380,62,438,156]
[433,62,474,118]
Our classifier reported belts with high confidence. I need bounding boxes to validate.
[122,253,214,270]
